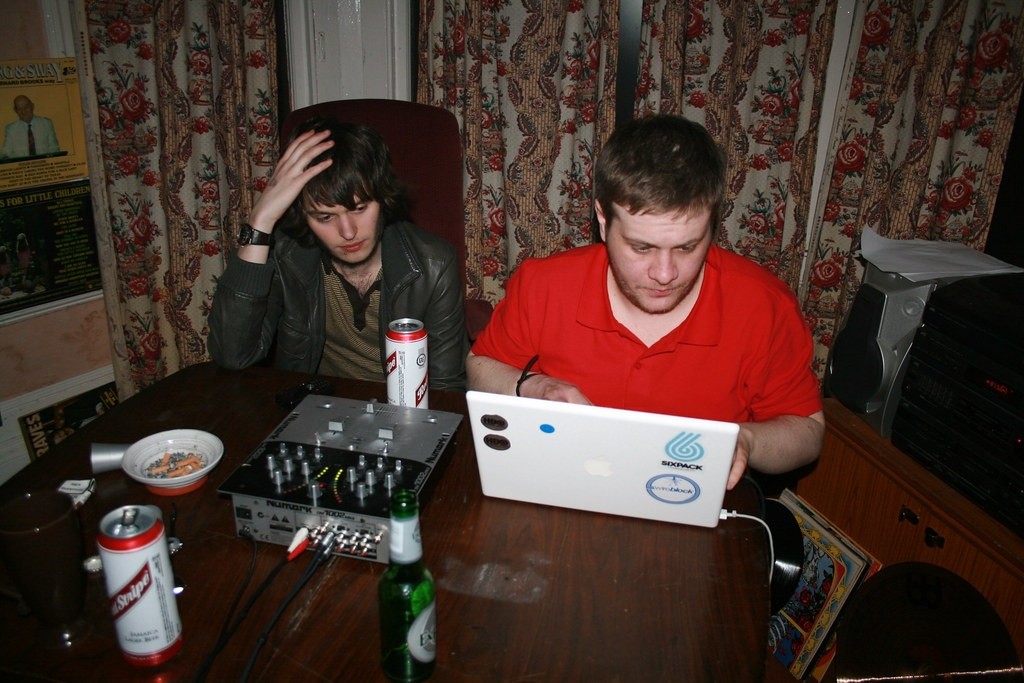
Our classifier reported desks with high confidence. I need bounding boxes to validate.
[0,361,771,683]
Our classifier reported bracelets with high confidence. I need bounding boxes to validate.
[516,354,541,397]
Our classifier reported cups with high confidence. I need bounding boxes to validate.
[0,492,96,650]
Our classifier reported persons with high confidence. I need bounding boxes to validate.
[2,95,61,159]
[208,119,465,392]
[466,112,827,491]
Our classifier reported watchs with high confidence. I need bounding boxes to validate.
[236,223,276,248]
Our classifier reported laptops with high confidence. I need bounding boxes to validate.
[466,390,740,527]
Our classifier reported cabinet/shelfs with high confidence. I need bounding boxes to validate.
[798,399,1024,666]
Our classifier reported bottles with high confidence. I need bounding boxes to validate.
[379,490,436,683]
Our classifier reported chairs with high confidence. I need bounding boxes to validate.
[277,98,820,622]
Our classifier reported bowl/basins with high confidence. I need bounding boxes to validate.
[121,429,224,489]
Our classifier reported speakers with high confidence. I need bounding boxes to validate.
[824,263,954,440]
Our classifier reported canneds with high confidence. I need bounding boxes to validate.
[385,318,430,410]
[97,504,183,667]
